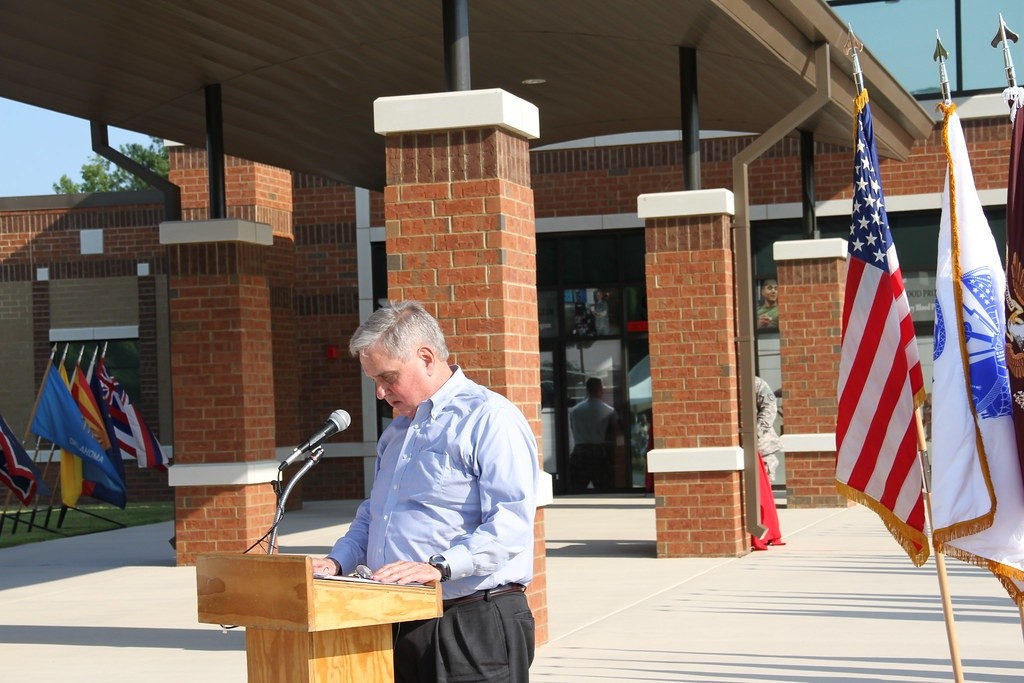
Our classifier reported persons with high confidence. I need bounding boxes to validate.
[311,297,539,683]
[754,376,782,484]
[570,378,617,491]
[755,271,778,330]
[591,291,609,335]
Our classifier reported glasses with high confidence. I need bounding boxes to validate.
[353,564,373,579]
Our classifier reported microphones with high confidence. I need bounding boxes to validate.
[279,409,351,470]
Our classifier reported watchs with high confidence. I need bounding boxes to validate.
[430,553,451,583]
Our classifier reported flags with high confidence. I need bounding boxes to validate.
[833,87,928,568]
[0,413,41,507]
[30,358,171,510]
[931,87,1024,637]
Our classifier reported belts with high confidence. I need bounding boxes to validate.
[443,582,523,608]
